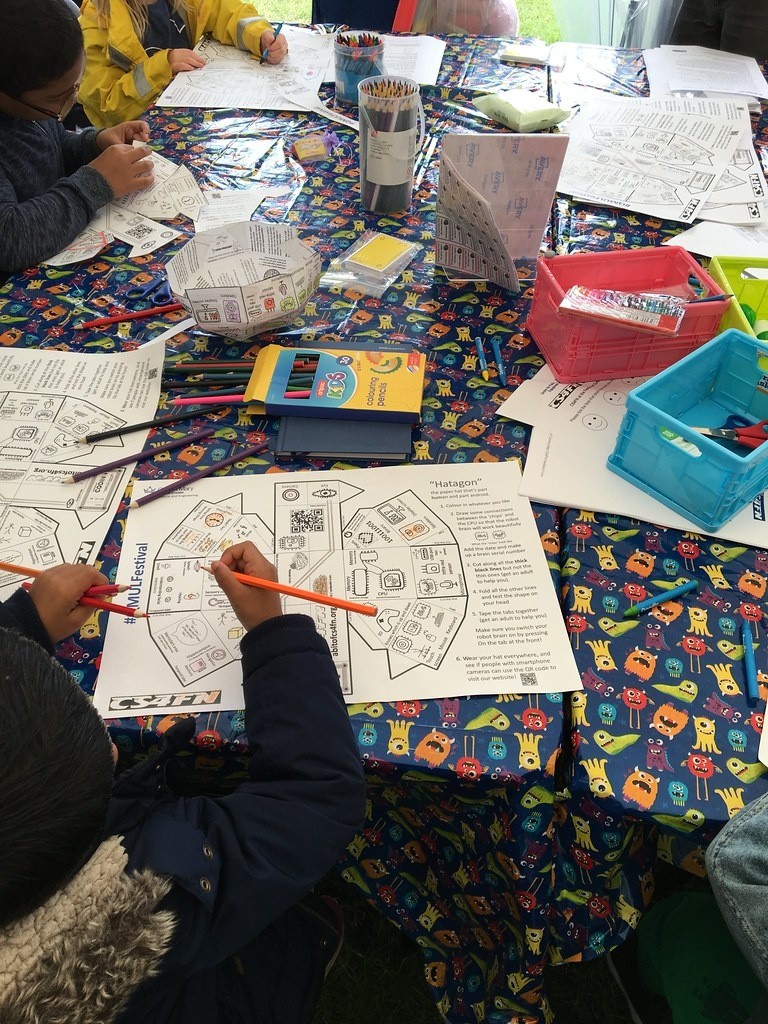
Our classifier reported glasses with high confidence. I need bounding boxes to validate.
[0,50,88,123]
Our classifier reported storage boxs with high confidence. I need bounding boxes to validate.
[706,254,768,375]
[605,327,768,533]
[527,244,734,385]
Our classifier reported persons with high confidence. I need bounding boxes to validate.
[0,0,288,282]
[0,541,369,1024]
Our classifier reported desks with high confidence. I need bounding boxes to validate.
[0,24,768,1024]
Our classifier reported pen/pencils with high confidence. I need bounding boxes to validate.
[358,78,417,215]
[73,405,226,443]
[61,429,217,484]
[69,303,183,329]
[0,562,131,601]
[690,294,735,303]
[21,583,151,618]
[161,353,319,406]
[201,566,378,617]
[125,442,269,509]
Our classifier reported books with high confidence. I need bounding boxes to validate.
[274,340,413,463]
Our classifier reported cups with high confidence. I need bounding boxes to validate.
[334,29,386,104]
[357,75,420,216]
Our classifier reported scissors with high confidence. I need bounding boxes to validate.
[125,273,173,307]
[692,415,768,448]
